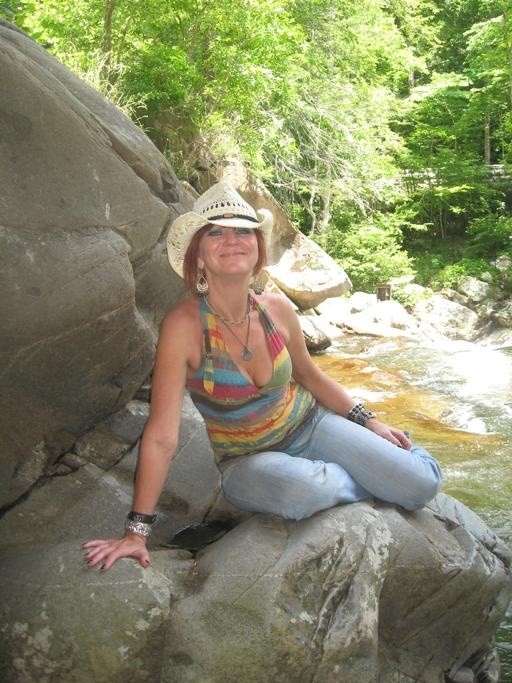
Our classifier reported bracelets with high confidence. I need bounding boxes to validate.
[126,508,157,524]
[124,519,153,537]
[347,403,375,426]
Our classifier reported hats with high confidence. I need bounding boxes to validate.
[166,182,274,280]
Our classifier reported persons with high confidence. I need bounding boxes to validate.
[81,178,443,572]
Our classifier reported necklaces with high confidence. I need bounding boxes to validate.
[218,315,257,361]
[200,291,252,325]
